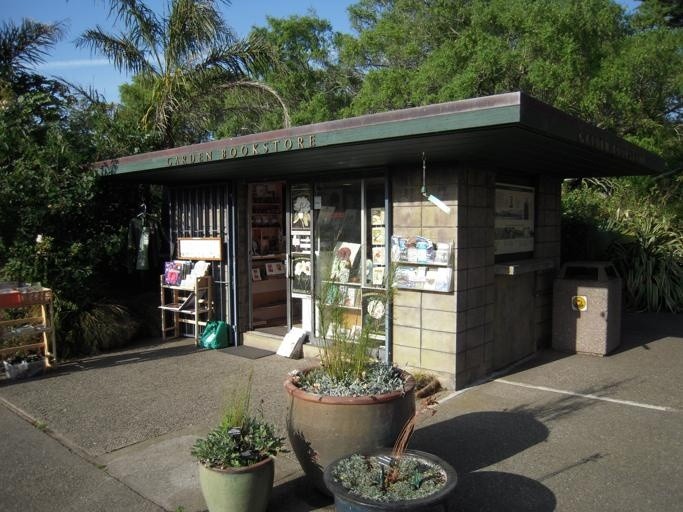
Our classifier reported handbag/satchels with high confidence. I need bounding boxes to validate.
[198,321,231,350]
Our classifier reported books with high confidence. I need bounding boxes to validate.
[162,259,210,309]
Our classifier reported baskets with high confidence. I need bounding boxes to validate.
[21,285,52,306]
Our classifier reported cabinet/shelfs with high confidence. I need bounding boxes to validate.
[0,282,56,380]
[156,272,212,348]
[248,182,287,260]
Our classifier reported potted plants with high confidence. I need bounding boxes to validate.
[319,397,458,512]
[283,261,417,510]
[188,366,291,511]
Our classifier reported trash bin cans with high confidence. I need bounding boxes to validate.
[552,261,622,358]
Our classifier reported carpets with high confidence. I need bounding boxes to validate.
[220,344,277,360]
